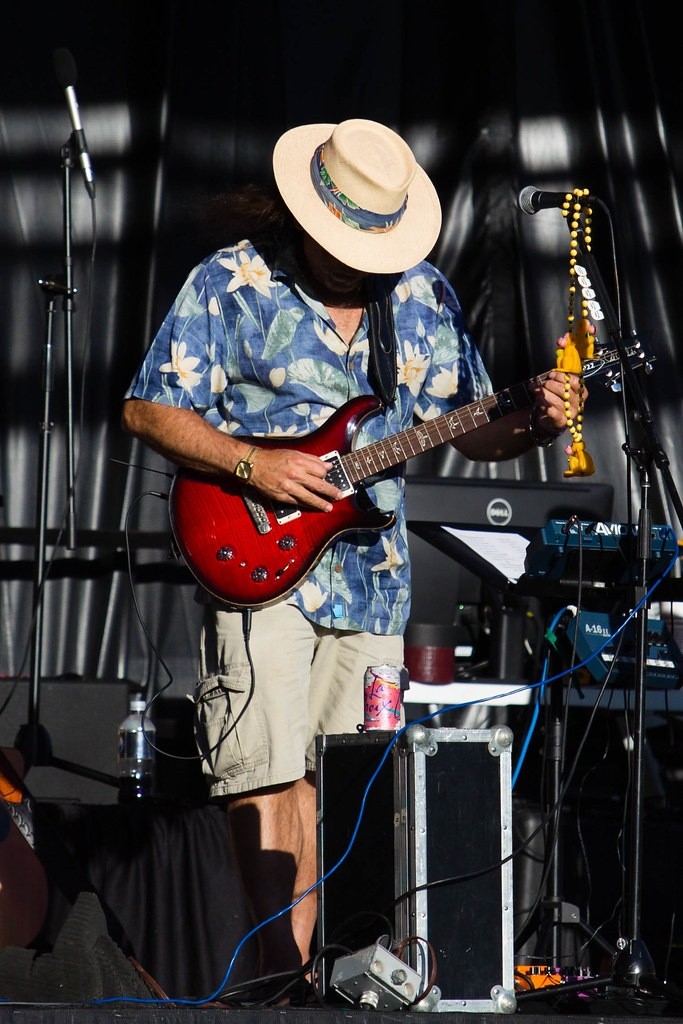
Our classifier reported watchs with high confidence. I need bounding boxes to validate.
[234,446,262,483]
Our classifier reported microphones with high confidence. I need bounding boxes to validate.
[517,186,595,215]
[65,85,96,201]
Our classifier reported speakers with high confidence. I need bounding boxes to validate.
[0,676,143,807]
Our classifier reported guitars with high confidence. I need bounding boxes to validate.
[165,332,656,614]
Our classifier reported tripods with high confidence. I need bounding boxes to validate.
[514,213,683,1000]
[11,143,121,788]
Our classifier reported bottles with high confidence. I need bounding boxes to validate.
[117,701,156,803]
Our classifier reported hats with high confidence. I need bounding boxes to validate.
[272,119,442,274]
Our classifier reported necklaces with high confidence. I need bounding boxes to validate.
[560,186,597,479]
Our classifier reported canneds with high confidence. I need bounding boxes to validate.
[363,663,402,731]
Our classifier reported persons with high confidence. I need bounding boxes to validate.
[120,121,589,1003]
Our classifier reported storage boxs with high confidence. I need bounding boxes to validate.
[314,724,516,1014]
